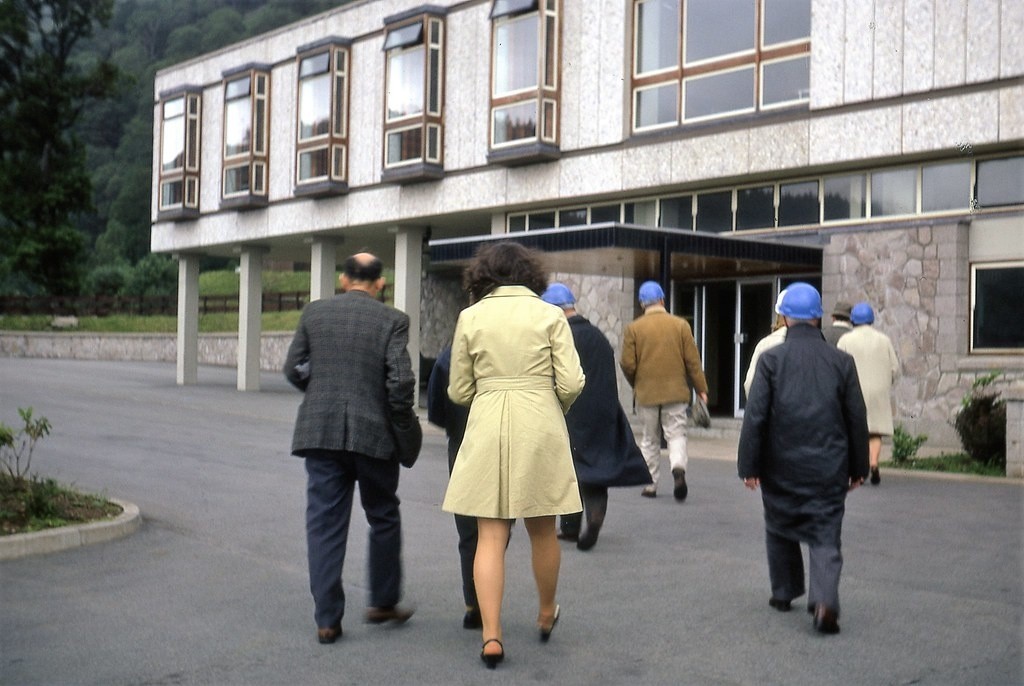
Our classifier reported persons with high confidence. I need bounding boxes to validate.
[837,303,902,485]
[738,283,869,633]
[540,283,653,551]
[742,289,788,399]
[821,302,856,347]
[620,280,708,502]
[281,253,422,646]
[427,344,515,630]
[442,242,585,670]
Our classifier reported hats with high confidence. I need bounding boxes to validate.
[832,302,852,318]
[692,395,710,429]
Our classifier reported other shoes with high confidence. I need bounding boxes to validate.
[673,467,688,500]
[557,531,578,542]
[577,516,601,550]
[368,606,414,624]
[642,488,656,498]
[816,607,839,633]
[871,467,881,485]
[318,623,341,643]
[768,597,790,612]
[463,611,482,629]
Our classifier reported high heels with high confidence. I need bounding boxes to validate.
[481,639,504,669]
[537,604,560,640]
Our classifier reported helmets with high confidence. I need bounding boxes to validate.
[539,284,575,304]
[775,282,823,319]
[851,303,874,325]
[638,281,664,301]
